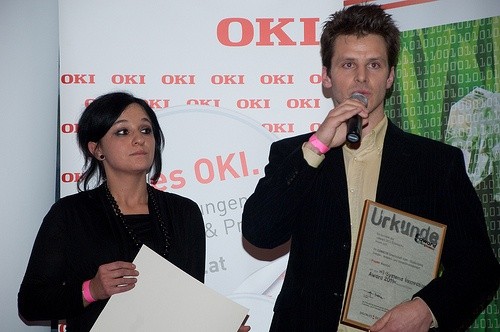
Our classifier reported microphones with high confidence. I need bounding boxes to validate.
[347,93,367,144]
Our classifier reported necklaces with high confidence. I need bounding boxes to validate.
[103,180,172,259]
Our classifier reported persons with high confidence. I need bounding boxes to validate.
[17,92,251,332]
[241,4,500,332]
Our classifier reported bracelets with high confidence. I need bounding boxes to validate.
[81,279,97,304]
[309,133,330,155]
